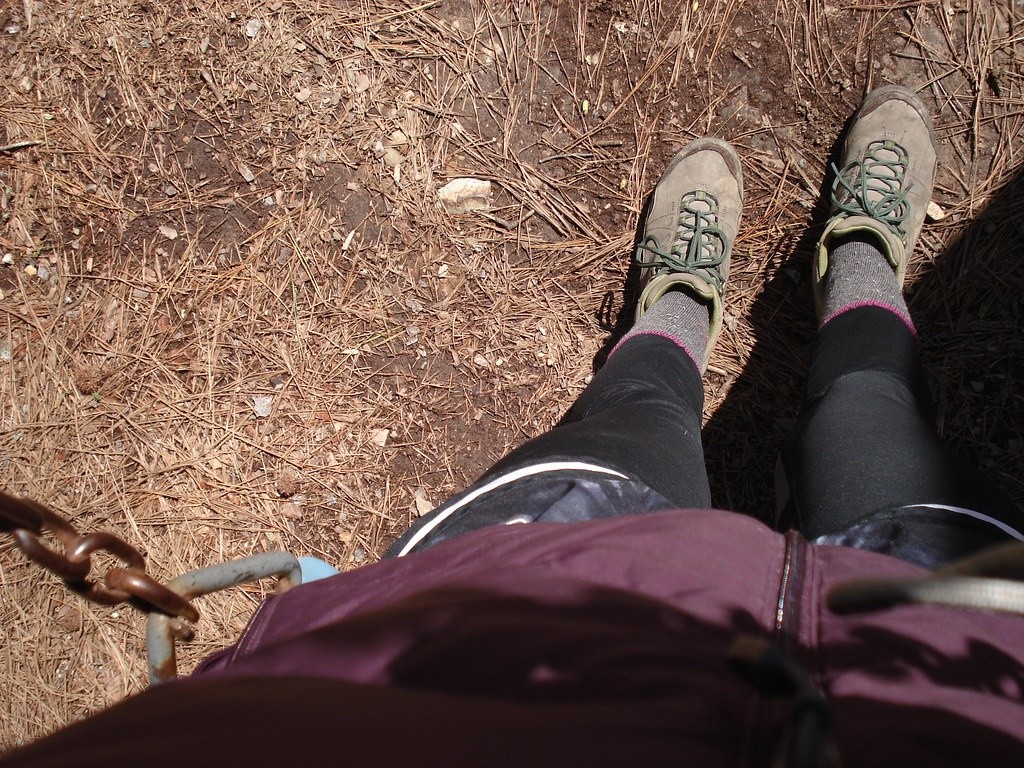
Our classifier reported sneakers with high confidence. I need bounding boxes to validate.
[634,136,743,377]
[812,84,937,319]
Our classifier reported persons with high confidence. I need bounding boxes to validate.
[0,85,1024,767]
